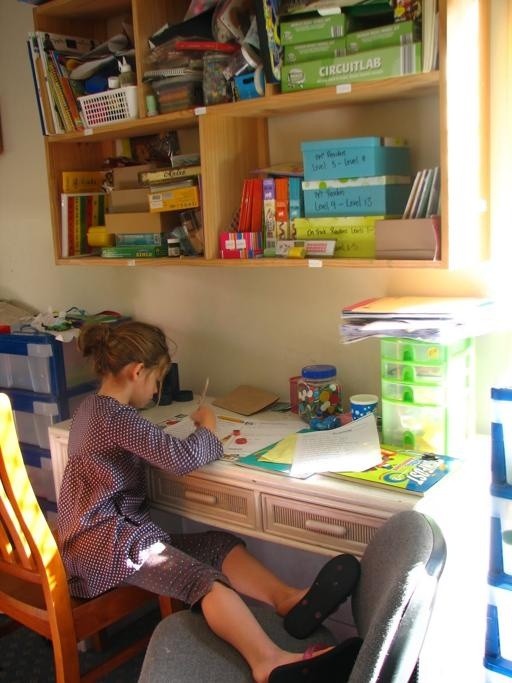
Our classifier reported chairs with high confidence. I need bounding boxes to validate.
[0,391,186,681]
[136,510,447,682]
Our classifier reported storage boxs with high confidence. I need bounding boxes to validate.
[292,135,438,260]
[0,310,103,515]
[379,336,479,460]
[482,385,512,683]
[152,76,202,112]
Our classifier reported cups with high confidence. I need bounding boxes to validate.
[350,394,377,437]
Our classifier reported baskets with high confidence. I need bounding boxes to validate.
[77,85,138,127]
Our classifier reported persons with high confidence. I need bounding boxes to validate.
[54,319,364,682]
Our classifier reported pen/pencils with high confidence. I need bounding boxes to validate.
[216,415,244,423]
[198,377,210,411]
[157,85,193,114]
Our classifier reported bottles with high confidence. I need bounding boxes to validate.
[299,365,344,421]
[202,50,233,104]
[106,63,138,119]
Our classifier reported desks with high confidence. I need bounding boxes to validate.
[48,392,447,653]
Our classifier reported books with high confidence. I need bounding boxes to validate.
[338,293,491,347]
[24,30,103,137]
[221,161,303,262]
[402,164,440,220]
[316,441,460,497]
[235,427,320,480]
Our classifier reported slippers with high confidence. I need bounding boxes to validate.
[282,552,361,638]
[267,636,364,683]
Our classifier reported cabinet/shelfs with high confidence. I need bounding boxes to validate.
[33,0,484,275]
